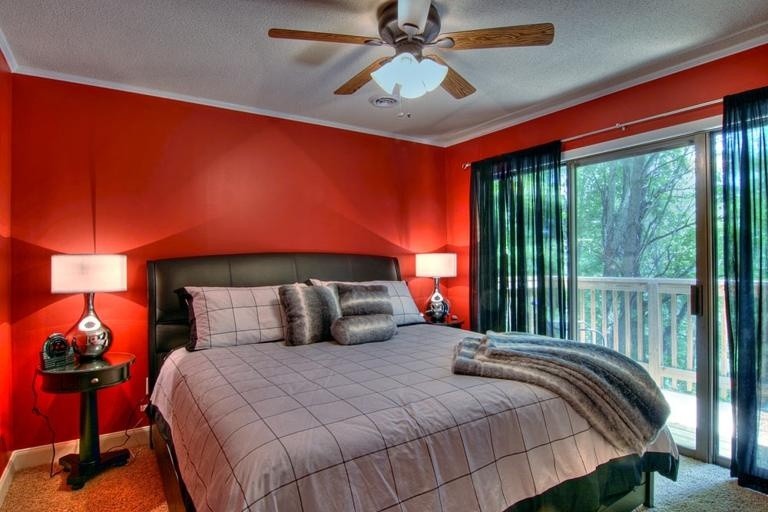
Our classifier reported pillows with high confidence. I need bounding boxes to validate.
[175,280,425,350]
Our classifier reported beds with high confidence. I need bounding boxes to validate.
[145,252,654,510]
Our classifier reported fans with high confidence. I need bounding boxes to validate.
[268,1,555,101]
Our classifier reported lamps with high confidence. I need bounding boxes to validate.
[50,253,127,360]
[415,254,457,321]
[372,1,449,99]
